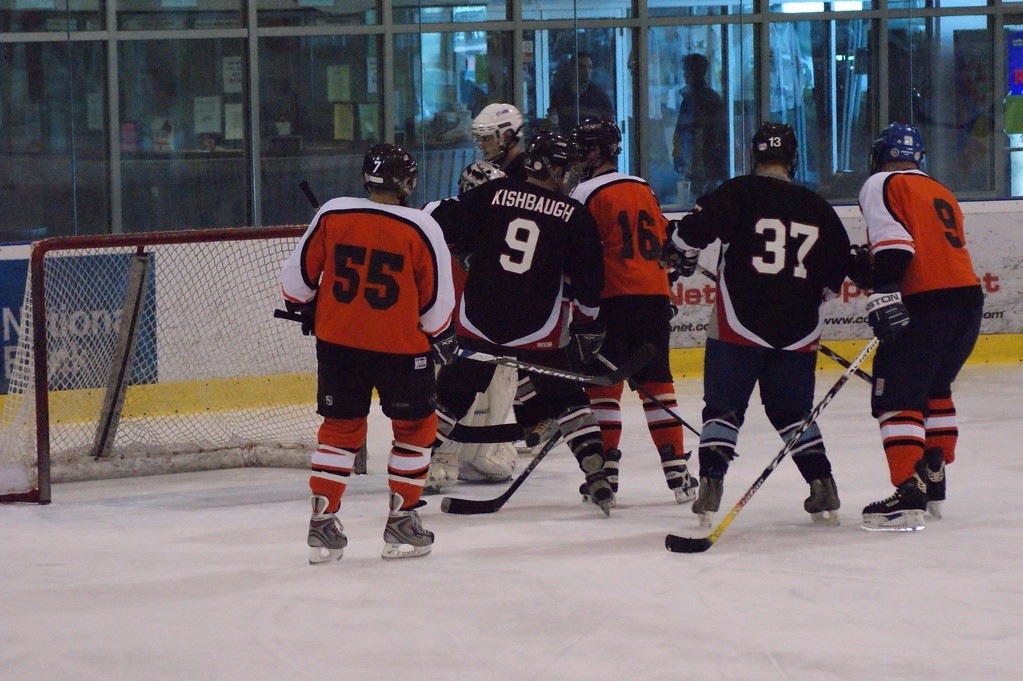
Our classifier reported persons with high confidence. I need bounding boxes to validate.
[441,51,488,120]
[672,53,730,196]
[280,105,699,547]
[857,122,985,517]
[663,120,850,513]
[557,51,613,133]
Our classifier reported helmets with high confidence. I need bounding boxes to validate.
[870,121,926,174]
[363,143,418,206]
[458,159,510,198]
[524,128,583,198]
[570,115,622,183]
[749,120,800,180]
[471,102,524,168]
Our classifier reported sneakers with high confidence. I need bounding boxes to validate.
[526,415,566,457]
[306,494,348,564]
[381,488,434,559]
[692,476,723,529]
[861,460,928,533]
[923,445,946,519]
[658,442,699,504]
[579,447,622,504]
[579,449,616,517]
[803,473,841,527]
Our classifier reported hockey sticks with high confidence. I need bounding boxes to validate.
[273,308,658,387]
[296,177,536,446]
[686,260,876,386]
[666,331,884,551]
[439,271,680,516]
[591,350,705,437]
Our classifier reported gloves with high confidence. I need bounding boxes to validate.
[837,243,871,290]
[429,323,460,366]
[866,289,914,346]
[568,315,608,365]
[657,220,701,277]
[284,296,315,336]
